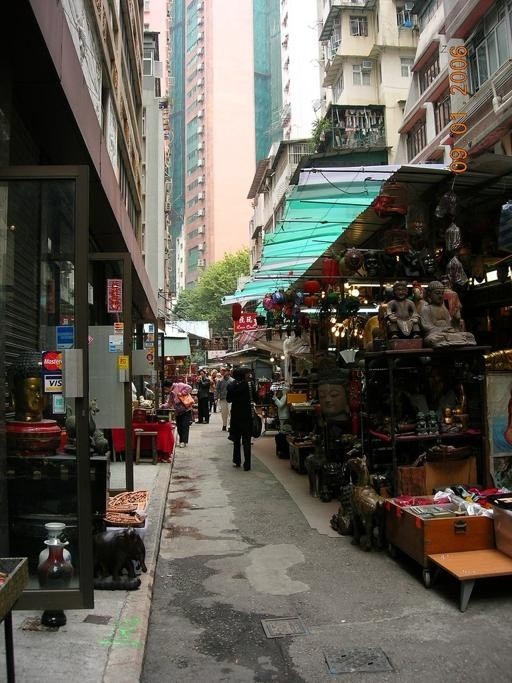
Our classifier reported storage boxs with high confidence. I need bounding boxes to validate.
[397,455,477,497]
[382,498,494,589]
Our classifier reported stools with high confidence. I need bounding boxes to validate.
[133,431,159,464]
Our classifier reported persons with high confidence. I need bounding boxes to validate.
[7,348,46,421]
[384,280,421,340]
[226,367,254,471]
[315,357,350,422]
[441,277,467,333]
[420,279,477,349]
[411,279,427,316]
[145,363,316,448]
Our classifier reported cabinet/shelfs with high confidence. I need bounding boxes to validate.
[362,345,491,498]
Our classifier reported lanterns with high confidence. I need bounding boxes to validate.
[232,302,242,321]
[262,277,321,340]
[321,257,337,286]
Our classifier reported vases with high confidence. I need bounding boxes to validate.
[37,521,74,627]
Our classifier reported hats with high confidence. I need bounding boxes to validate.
[222,367,231,372]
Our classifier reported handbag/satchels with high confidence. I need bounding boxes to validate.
[251,407,263,439]
[177,393,195,409]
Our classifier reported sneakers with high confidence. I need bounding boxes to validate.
[232,464,251,472]
[179,440,187,449]
[195,420,210,425]
[222,425,227,432]
